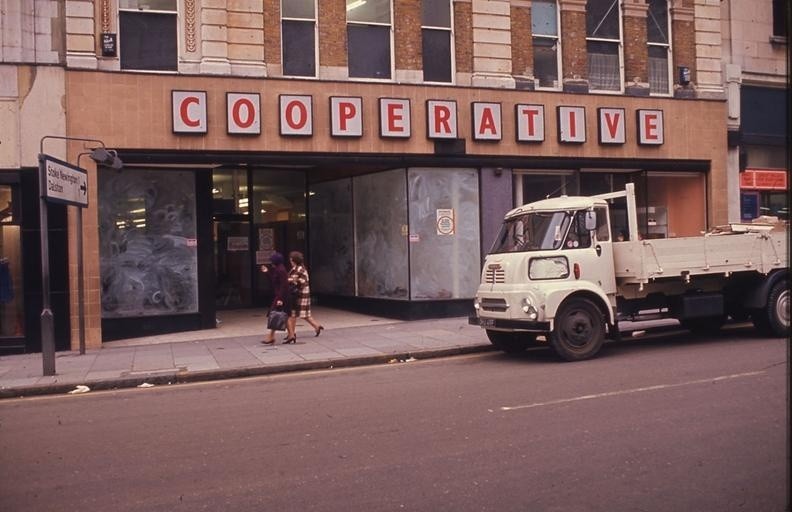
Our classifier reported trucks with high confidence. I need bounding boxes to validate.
[469,178,791,363]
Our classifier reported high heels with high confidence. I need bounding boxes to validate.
[315,325,324,336]
[283,333,297,339]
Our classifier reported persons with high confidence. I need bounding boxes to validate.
[259,252,296,344]
[283,250,325,340]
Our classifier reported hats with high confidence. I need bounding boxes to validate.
[268,254,284,262]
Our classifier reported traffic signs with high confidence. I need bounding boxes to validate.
[43,155,90,207]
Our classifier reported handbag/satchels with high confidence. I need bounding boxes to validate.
[267,309,288,331]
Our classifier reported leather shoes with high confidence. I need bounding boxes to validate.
[282,336,295,343]
[260,339,275,344]
